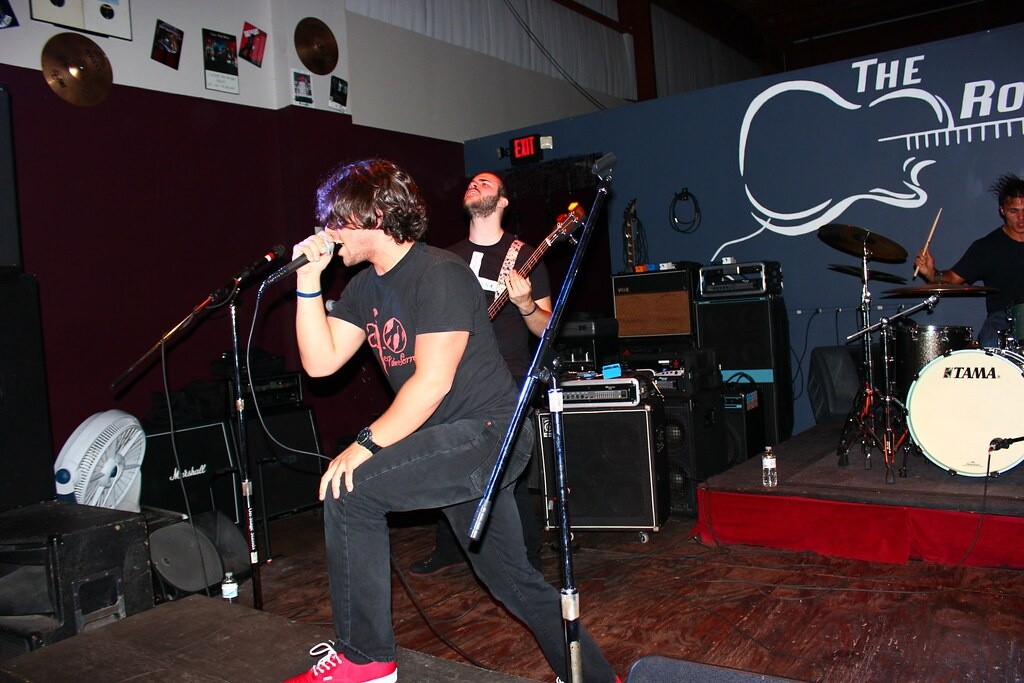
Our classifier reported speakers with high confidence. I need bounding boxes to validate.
[613,268,699,339]
[145,505,253,604]
[140,416,248,530]
[720,389,767,470]
[808,345,890,425]
[693,293,796,445]
[644,381,727,517]
[219,405,328,525]
[535,401,671,532]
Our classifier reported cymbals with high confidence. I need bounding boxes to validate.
[879,285,999,295]
[817,223,909,263]
[826,263,909,282]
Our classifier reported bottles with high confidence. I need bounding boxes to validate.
[221,572,239,605]
[762,446,777,487]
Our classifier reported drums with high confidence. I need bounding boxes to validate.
[1004,293,1024,359]
[902,346,1023,479]
[892,323,973,403]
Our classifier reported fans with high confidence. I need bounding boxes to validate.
[53,409,146,515]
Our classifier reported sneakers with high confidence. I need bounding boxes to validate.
[409,548,470,577]
[283,639,398,683]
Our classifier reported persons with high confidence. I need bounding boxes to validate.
[282,156,624,683]
[409,172,552,578]
[914,178,1024,349]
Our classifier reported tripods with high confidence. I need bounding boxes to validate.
[832,246,940,485]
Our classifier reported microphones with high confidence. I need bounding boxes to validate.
[268,241,335,285]
[242,244,286,278]
[989,438,1003,450]
[326,300,337,311]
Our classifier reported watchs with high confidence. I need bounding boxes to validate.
[356,426,382,455]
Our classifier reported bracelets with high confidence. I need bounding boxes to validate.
[933,269,942,281]
[296,289,323,298]
[521,304,537,316]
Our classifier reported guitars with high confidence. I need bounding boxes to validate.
[486,201,593,319]
